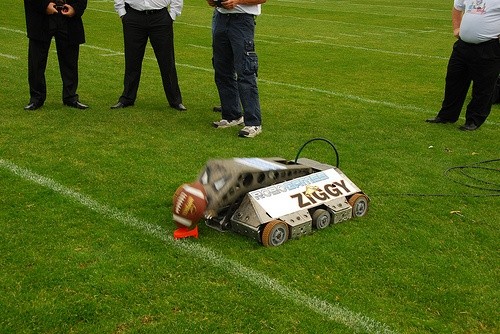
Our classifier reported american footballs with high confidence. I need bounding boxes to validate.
[173,181,209,228]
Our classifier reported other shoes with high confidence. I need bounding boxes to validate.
[213,105,222,112]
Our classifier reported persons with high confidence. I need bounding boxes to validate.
[110,0,187,111]
[23,0,88,110]
[426,0,500,130]
[206,0,267,137]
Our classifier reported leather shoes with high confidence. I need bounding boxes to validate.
[426,118,447,123]
[175,103,187,111]
[67,101,89,109]
[110,101,125,109]
[24,102,39,110]
[460,123,477,131]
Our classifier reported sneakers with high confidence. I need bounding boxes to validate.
[213,116,243,128]
[238,124,262,137]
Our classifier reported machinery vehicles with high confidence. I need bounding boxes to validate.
[192,155,370,250]
